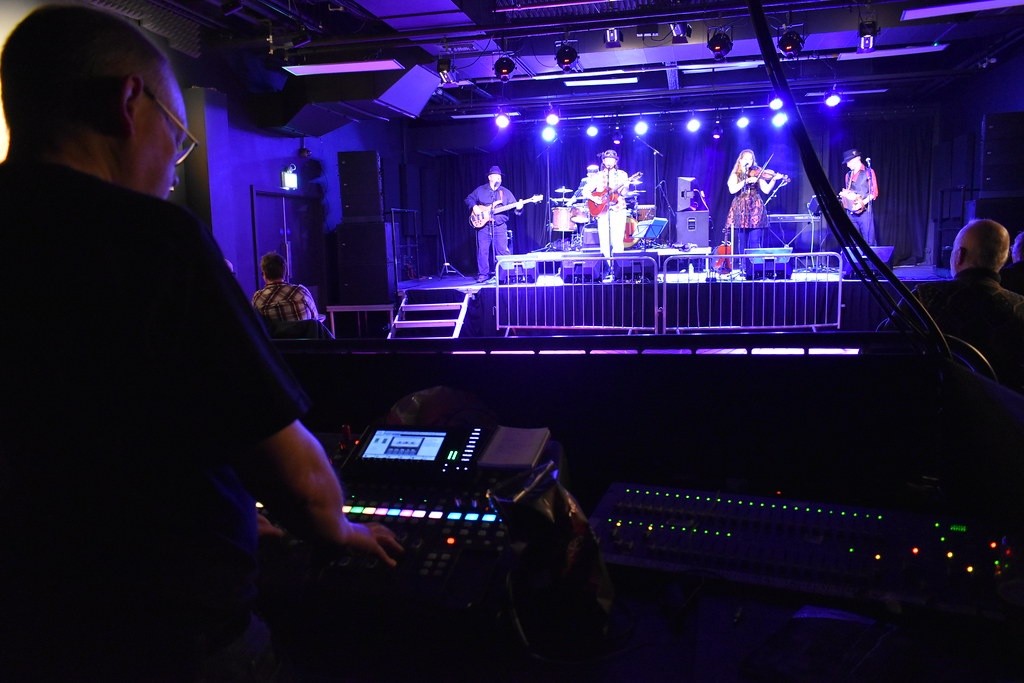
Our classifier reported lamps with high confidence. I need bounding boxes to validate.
[857,9,881,49]
[633,115,650,138]
[685,107,703,133]
[439,57,458,86]
[769,95,783,111]
[549,37,585,73]
[774,24,806,60]
[708,106,725,142]
[704,24,735,61]
[493,106,512,129]
[824,84,845,108]
[585,116,598,137]
[735,107,750,129]
[546,104,562,126]
[606,112,624,146]
[490,51,518,84]
[600,27,625,48]
[669,22,694,43]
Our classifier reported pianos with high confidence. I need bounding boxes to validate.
[765,214,822,270]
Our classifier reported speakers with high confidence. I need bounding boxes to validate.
[560,253,610,283]
[744,248,794,280]
[496,255,539,284]
[840,246,895,279]
[334,151,403,305]
[612,252,659,280]
[963,112,1024,246]
[676,176,710,247]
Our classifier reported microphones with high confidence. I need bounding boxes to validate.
[608,164,611,169]
[556,133,562,143]
[656,180,664,189]
[633,134,638,142]
[866,157,871,167]
[743,163,749,167]
[494,182,500,187]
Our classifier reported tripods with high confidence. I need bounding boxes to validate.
[531,137,563,253]
[435,207,466,281]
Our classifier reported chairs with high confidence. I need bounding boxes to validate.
[271,320,334,339]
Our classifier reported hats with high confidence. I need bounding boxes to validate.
[487,166,505,177]
[841,148,862,164]
[602,149,620,161]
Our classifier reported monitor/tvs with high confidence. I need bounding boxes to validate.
[354,426,453,467]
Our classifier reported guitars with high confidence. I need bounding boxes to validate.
[469,194,544,228]
[588,171,642,216]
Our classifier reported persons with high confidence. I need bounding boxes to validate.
[0,3,405,683]
[725,149,783,271]
[583,150,628,282]
[574,165,599,203]
[839,149,878,246]
[252,252,318,329]
[999,232,1024,296]
[865,218,1024,394]
[464,166,523,283]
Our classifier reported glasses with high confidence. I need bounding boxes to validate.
[140,80,199,166]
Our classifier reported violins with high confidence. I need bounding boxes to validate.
[748,165,791,183]
[712,227,733,274]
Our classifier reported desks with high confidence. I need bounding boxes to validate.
[325,301,399,340]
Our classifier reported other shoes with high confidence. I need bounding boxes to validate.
[476,273,490,283]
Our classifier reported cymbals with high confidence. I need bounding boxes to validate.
[629,189,646,193]
[629,181,643,184]
[555,188,573,193]
[576,196,587,199]
[551,198,573,202]
[578,187,584,190]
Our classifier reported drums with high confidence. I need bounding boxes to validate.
[551,203,591,232]
[622,216,639,248]
[634,205,656,220]
